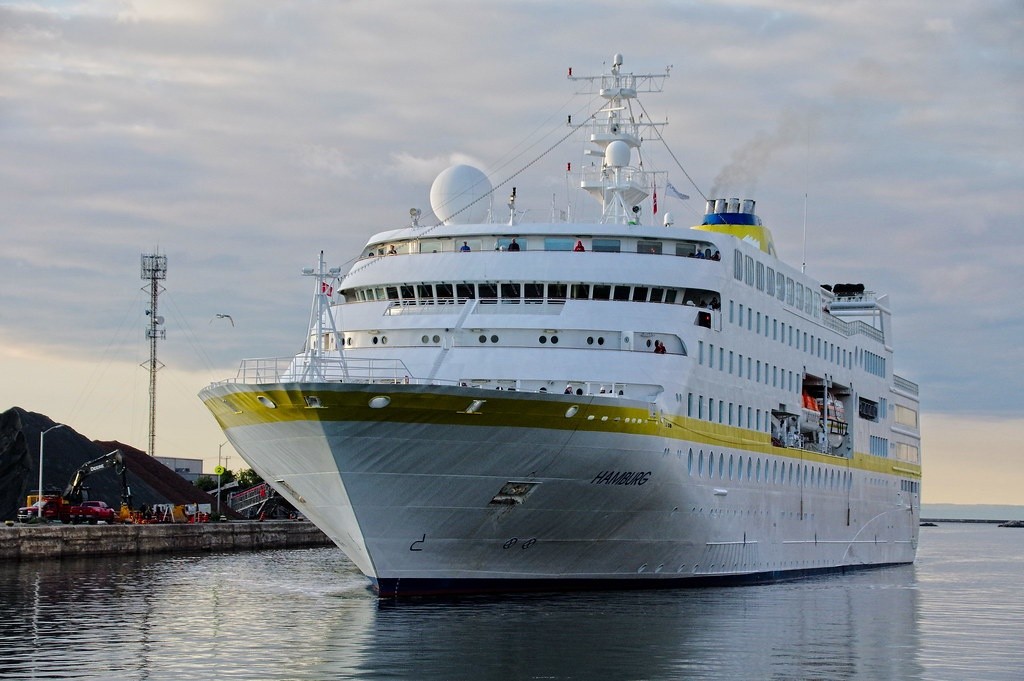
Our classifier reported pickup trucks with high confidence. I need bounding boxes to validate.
[70,501,117,524]
[17,496,71,524]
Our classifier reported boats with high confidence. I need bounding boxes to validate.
[197,48,923,596]
[799,395,820,432]
[820,401,846,443]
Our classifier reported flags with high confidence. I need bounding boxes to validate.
[653,184,658,214]
[665,180,689,200]
[323,282,332,296]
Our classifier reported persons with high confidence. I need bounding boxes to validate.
[508,238,519,251]
[700,297,720,309]
[388,244,397,254]
[660,343,666,354]
[687,247,720,261]
[563,384,573,395]
[260,482,270,500]
[574,240,584,251]
[138,503,171,523]
[599,387,605,394]
[494,240,505,251]
[459,241,471,252]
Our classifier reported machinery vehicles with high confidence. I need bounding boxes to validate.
[28,448,134,524]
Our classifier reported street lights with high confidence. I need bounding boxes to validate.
[38,425,64,519]
